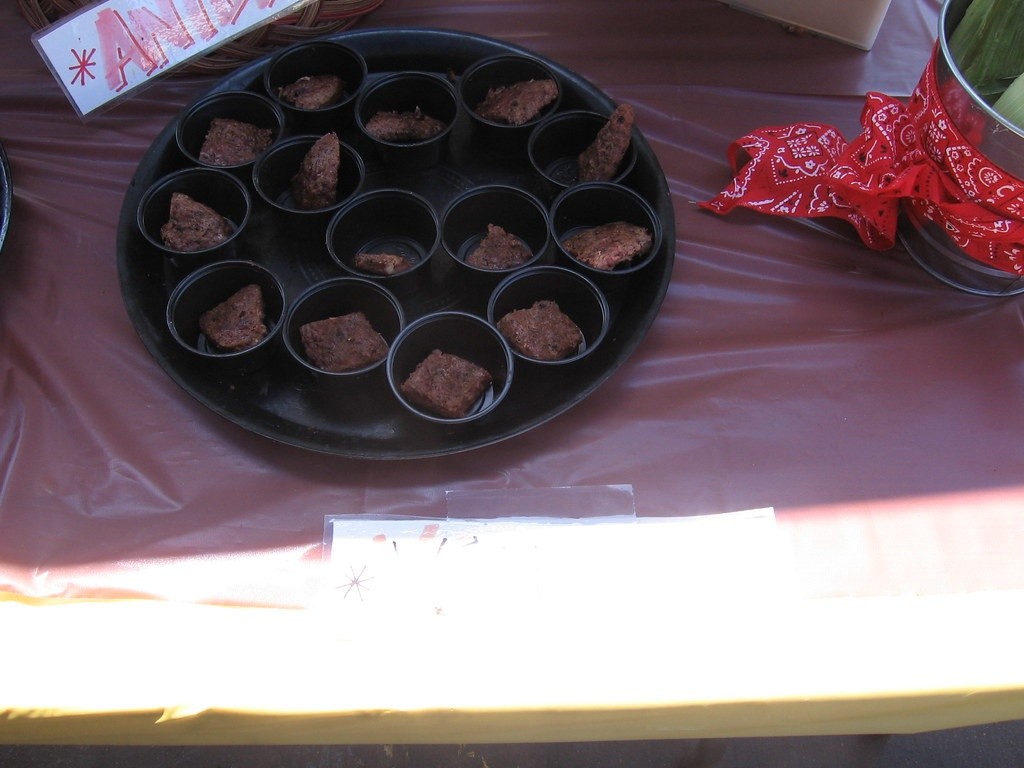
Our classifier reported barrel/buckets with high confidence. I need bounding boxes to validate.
[898,0,1024,295]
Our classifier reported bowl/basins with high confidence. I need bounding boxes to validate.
[253,136,364,226]
[386,312,513,425]
[441,185,549,273]
[175,90,287,170]
[528,110,636,187]
[548,182,662,291]
[355,71,458,166]
[165,261,286,377]
[283,277,407,382]
[458,55,561,142]
[326,189,439,297]
[486,266,610,371]
[136,167,251,266]
[265,40,366,133]
